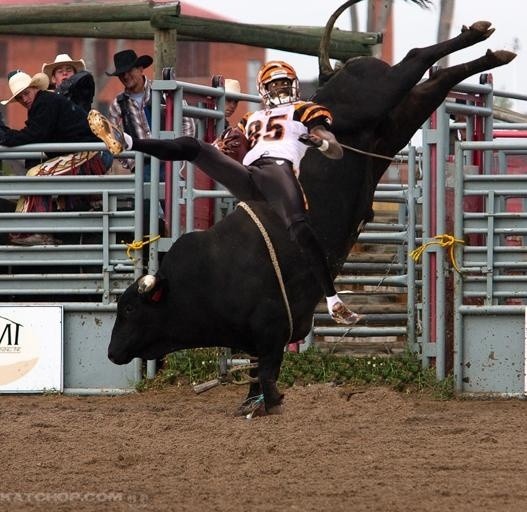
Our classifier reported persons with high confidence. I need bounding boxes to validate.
[0,50,241,245]
[87,61,359,325]
[449,98,474,155]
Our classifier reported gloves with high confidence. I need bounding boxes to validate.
[298,133,324,148]
[211,126,241,155]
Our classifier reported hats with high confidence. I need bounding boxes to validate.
[104,50,153,76]
[0,71,50,106]
[42,54,86,86]
[207,78,241,100]
[449,95,475,121]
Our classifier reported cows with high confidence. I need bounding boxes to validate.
[106,0,518,418]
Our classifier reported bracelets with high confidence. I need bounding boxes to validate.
[317,139,329,153]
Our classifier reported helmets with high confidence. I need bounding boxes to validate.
[257,61,301,110]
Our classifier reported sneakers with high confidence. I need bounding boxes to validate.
[330,301,360,326]
[86,110,129,156]
